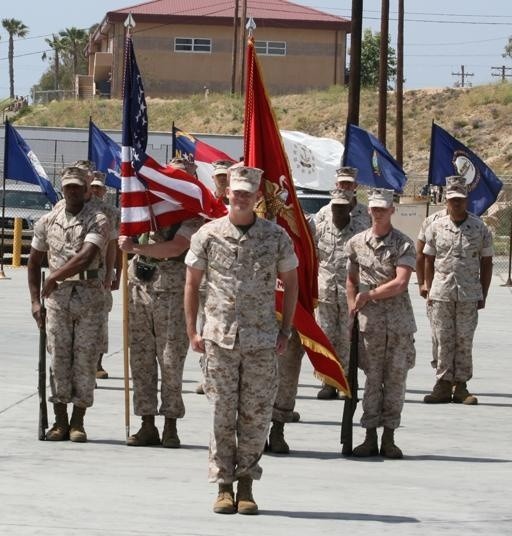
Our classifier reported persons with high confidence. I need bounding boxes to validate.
[119,159,204,447]
[211,164,232,202]
[29,159,123,441]
[344,187,419,460]
[414,176,494,405]
[181,164,302,517]
[259,167,374,456]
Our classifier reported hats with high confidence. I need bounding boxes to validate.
[228,167,264,194]
[367,187,395,209]
[444,176,468,200]
[211,159,235,176]
[73,160,95,175]
[336,165,359,182]
[59,166,87,188]
[89,171,107,188]
[167,157,196,171]
[330,188,358,206]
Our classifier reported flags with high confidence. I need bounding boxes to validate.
[241,35,353,401]
[339,118,410,200]
[1,117,59,208]
[171,122,241,168]
[425,117,504,217]
[115,32,231,237]
[85,116,121,190]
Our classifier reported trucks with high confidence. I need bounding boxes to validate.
[0,118,335,226]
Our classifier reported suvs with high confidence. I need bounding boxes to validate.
[1,183,64,255]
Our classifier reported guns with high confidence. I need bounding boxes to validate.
[38,272,48,442]
[342,312,360,454]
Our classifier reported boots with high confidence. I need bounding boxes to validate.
[425,378,452,403]
[455,381,478,405]
[380,426,403,457]
[45,402,88,442]
[354,426,378,455]
[91,349,108,378]
[128,413,160,445]
[318,374,350,400]
[162,416,181,447]
[212,471,259,514]
[268,418,290,454]
